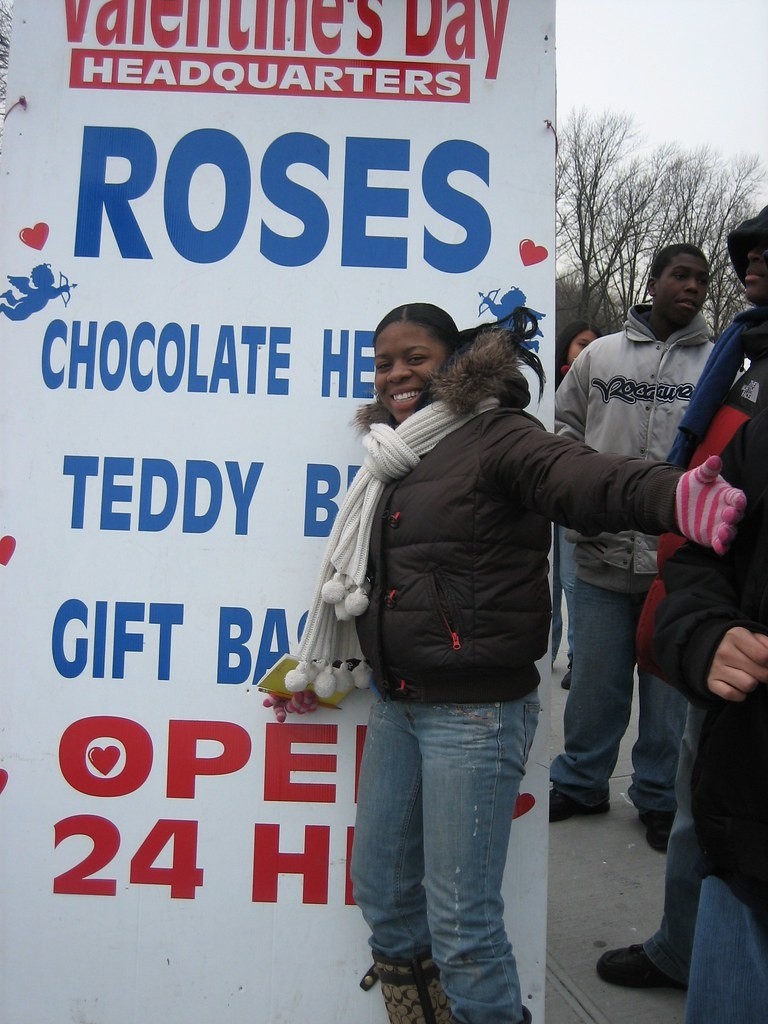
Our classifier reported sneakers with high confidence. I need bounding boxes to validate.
[549,789,611,823]
[638,809,675,853]
[596,944,689,991]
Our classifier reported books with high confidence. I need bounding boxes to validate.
[257,653,354,706]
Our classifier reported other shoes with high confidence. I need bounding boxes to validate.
[561,655,573,689]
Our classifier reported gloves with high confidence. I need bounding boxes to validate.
[262,689,319,723]
[673,454,747,556]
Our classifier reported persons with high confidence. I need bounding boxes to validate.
[549,207,767,1024]
[263,303,746,1024]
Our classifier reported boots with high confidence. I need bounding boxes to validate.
[359,949,460,1024]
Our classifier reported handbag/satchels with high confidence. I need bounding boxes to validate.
[634,531,689,687]
